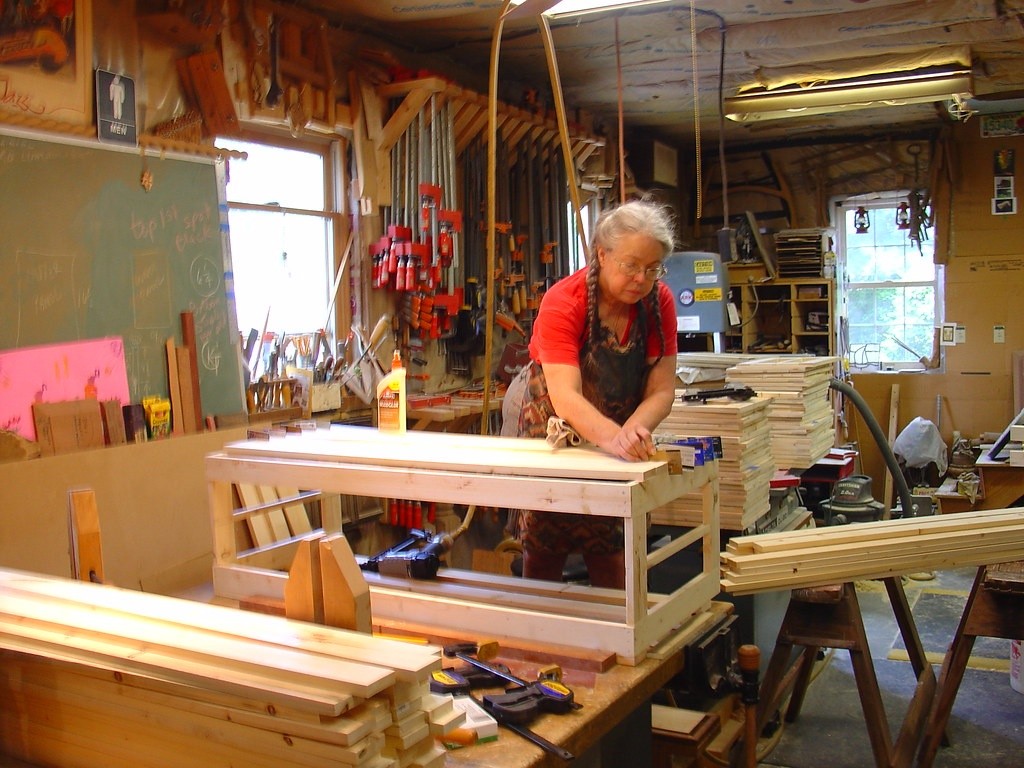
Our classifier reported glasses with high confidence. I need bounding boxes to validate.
[606,247,667,282]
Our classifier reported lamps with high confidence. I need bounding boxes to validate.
[853,192,870,234]
[896,186,912,229]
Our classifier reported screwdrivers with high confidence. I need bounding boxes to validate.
[319,355,343,382]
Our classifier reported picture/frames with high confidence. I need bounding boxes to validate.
[0,0,93,128]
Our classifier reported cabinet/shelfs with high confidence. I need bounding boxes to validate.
[706,278,847,448]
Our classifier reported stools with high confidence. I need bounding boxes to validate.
[753,575,951,768]
[915,560,1024,768]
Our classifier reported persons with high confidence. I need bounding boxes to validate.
[501,203,677,592]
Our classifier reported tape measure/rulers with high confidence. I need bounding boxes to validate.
[429,662,511,694]
[454,650,584,724]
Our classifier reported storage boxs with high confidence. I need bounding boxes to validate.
[31,397,170,458]
[728,262,767,284]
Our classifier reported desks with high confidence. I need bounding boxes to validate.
[975,450,1024,509]
[440,647,687,768]
